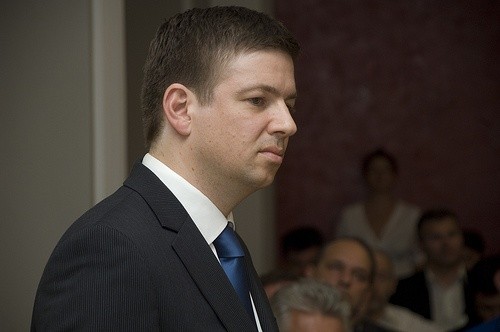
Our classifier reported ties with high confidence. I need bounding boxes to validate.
[214,225,258,332]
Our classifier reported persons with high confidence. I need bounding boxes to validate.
[32,5,301,332]
[260,145,500,332]
